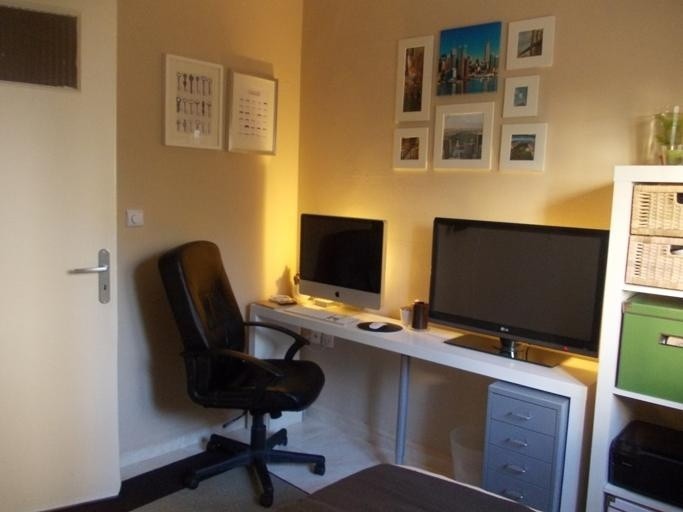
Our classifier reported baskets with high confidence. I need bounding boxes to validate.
[626,184,683,291]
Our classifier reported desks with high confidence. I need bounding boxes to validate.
[243,294,601,511]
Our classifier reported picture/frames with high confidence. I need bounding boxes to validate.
[161,52,223,152]
[392,34,436,123]
[390,127,429,174]
[499,120,550,173]
[502,74,540,120]
[226,66,280,159]
[505,15,557,72]
[431,98,496,176]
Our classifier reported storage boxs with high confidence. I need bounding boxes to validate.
[614,293,681,408]
[608,419,682,509]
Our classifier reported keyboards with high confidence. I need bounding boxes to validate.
[285,306,359,325]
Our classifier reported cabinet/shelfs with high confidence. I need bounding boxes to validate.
[480,375,572,511]
[578,162,682,511]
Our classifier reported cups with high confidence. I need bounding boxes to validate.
[645,104,682,166]
[399,305,411,324]
[411,301,429,330]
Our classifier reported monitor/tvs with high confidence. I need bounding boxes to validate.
[426,216,609,367]
[299,214,387,315]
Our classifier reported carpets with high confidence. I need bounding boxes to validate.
[285,456,533,512]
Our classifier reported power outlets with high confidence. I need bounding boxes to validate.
[320,332,336,350]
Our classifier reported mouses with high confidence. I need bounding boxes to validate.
[370,322,387,330]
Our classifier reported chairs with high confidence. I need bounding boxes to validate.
[160,238,332,507]
